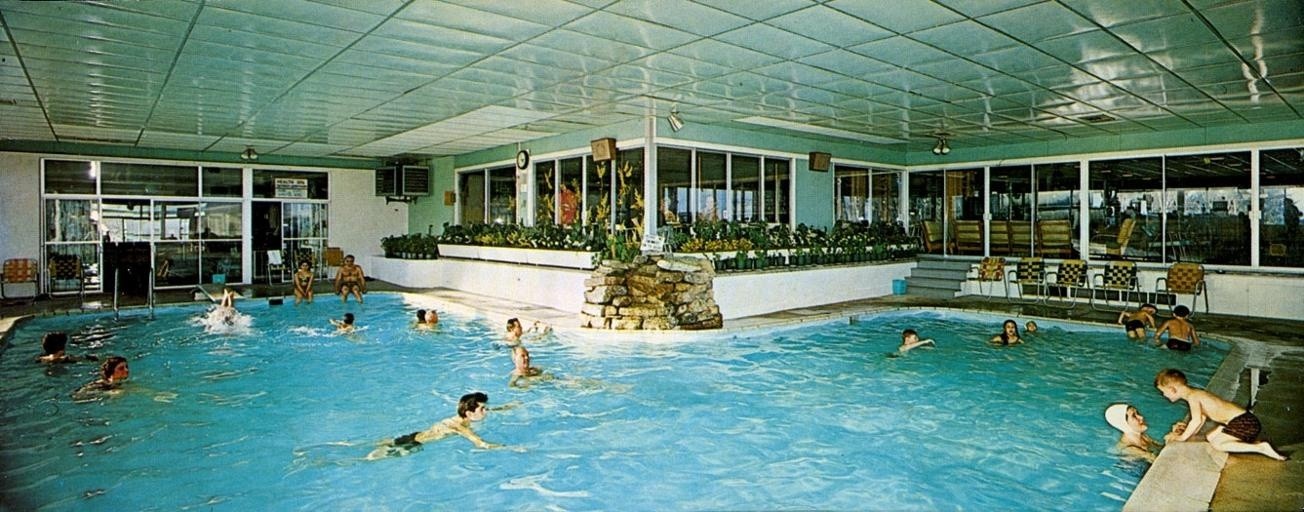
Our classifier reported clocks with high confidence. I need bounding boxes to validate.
[517,150,528,170]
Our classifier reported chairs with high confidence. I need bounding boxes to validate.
[0,248,345,307]
[920,218,1208,319]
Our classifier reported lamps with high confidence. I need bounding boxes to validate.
[240,149,258,160]
[666,114,685,133]
[932,134,951,155]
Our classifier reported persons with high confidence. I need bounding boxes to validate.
[289,391,531,473]
[1153,366,1288,462]
[1150,304,1200,353]
[204,289,236,334]
[416,309,438,330]
[292,258,314,307]
[1021,320,1041,336]
[71,355,162,405]
[1118,302,1157,343]
[1280,196,1299,241]
[410,309,425,331]
[696,194,722,221]
[504,346,637,398]
[1102,401,1188,464]
[500,317,553,346]
[35,331,100,366]
[876,327,936,363]
[324,312,371,347]
[333,253,368,305]
[984,319,1025,349]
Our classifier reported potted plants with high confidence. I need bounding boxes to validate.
[380,219,920,274]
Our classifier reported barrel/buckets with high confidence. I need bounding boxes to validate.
[892,279,908,295]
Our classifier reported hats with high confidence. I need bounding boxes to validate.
[1105,403,1130,433]
